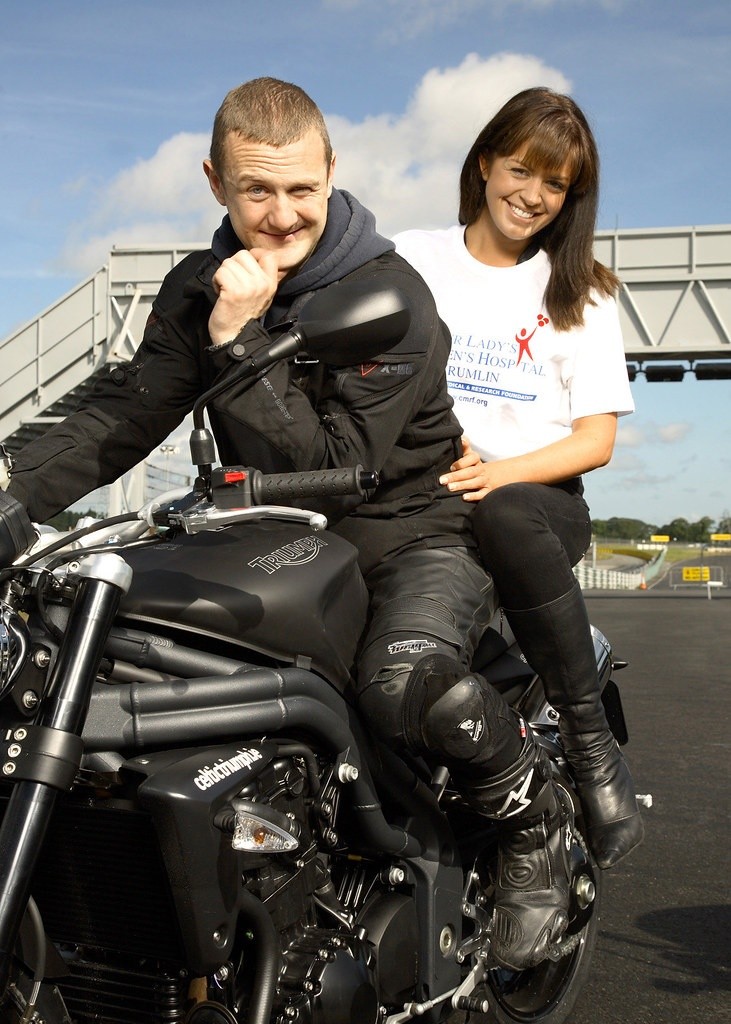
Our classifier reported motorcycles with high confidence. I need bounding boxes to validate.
[0,270,654,1024]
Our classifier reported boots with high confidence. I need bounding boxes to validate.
[451,709,576,972]
[499,579,646,870]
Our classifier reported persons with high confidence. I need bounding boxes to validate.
[1,78,581,974]
[382,85,646,871]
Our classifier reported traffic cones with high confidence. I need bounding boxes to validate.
[638,571,648,590]
